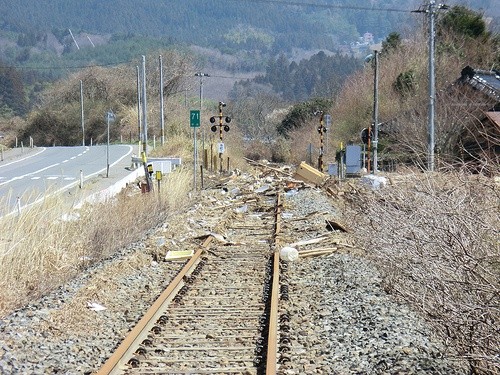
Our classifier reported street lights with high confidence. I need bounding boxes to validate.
[364,49,379,175]
